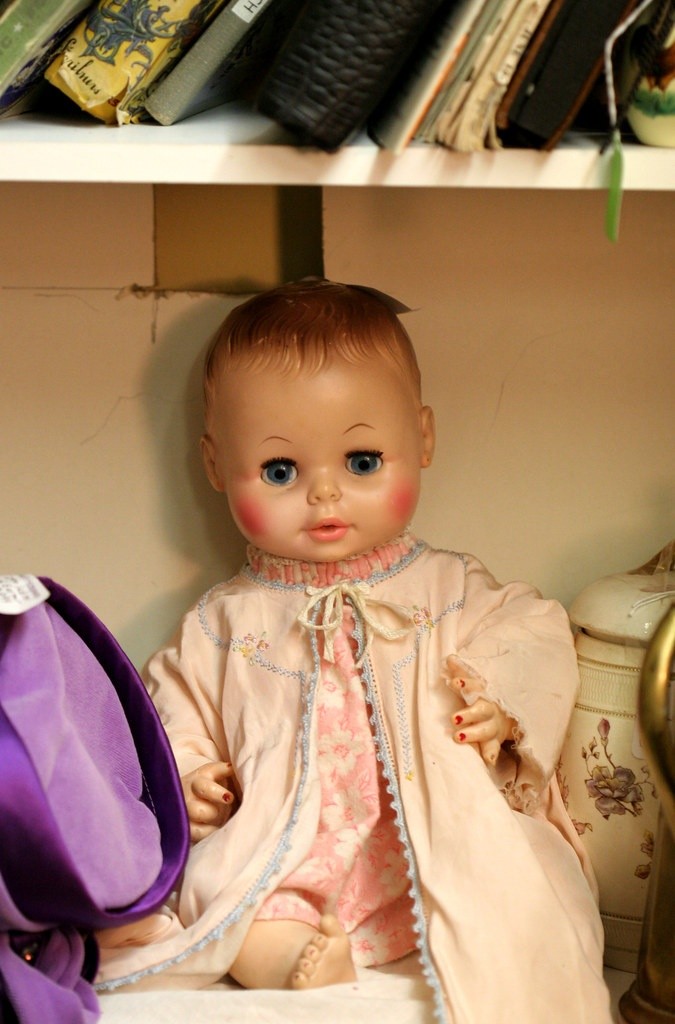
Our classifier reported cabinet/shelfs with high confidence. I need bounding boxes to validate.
[0,99,675,1024]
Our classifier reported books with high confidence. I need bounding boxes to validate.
[0,0,641,158]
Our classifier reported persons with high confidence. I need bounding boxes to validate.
[76,280,622,1024]
